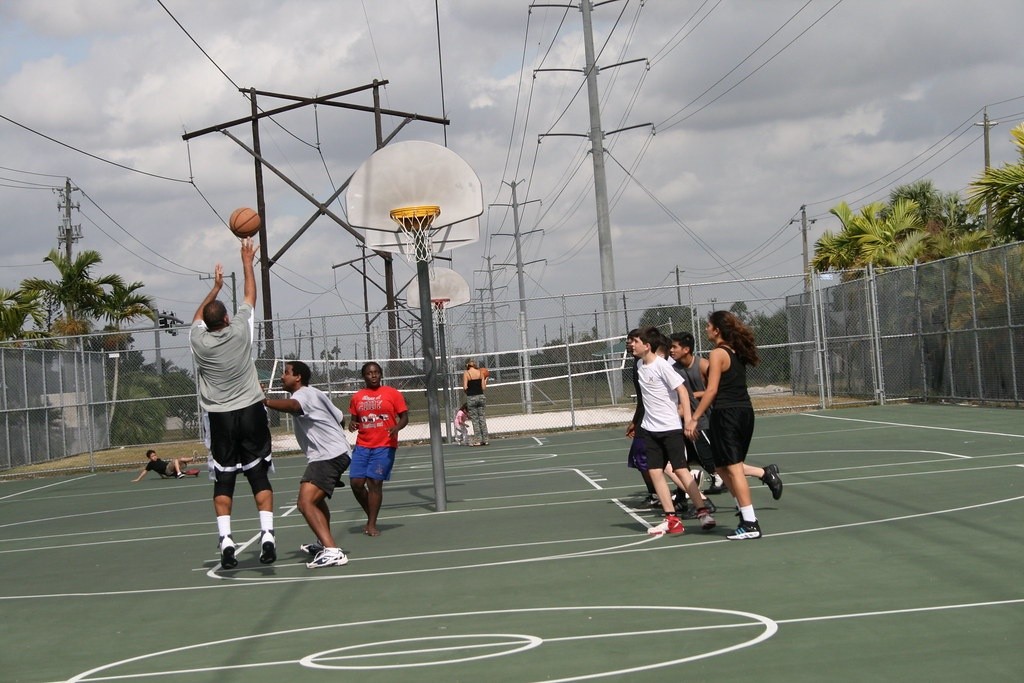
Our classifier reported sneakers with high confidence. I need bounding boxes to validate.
[217,534,238,569]
[306,546,349,569]
[735,510,744,527]
[650,514,683,535]
[691,470,703,489]
[759,465,782,500]
[637,493,662,508]
[258,530,277,564]
[727,520,762,540]
[703,481,728,495]
[681,497,716,520]
[300,540,325,555]
[696,510,715,531]
[672,492,690,500]
[673,498,688,512]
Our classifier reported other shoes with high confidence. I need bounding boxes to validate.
[455,438,461,445]
[485,442,488,445]
[177,473,185,478]
[465,443,468,445]
[193,450,198,462]
[474,443,481,445]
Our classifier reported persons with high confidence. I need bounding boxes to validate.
[464,357,491,445]
[188,237,276,570]
[260,361,351,569]
[131,450,198,482]
[454,402,473,448]
[348,362,409,537]
[625,310,782,540]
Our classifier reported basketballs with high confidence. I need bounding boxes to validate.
[229,207,262,238]
[479,368,490,380]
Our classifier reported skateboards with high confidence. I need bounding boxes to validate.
[170,469,200,477]
